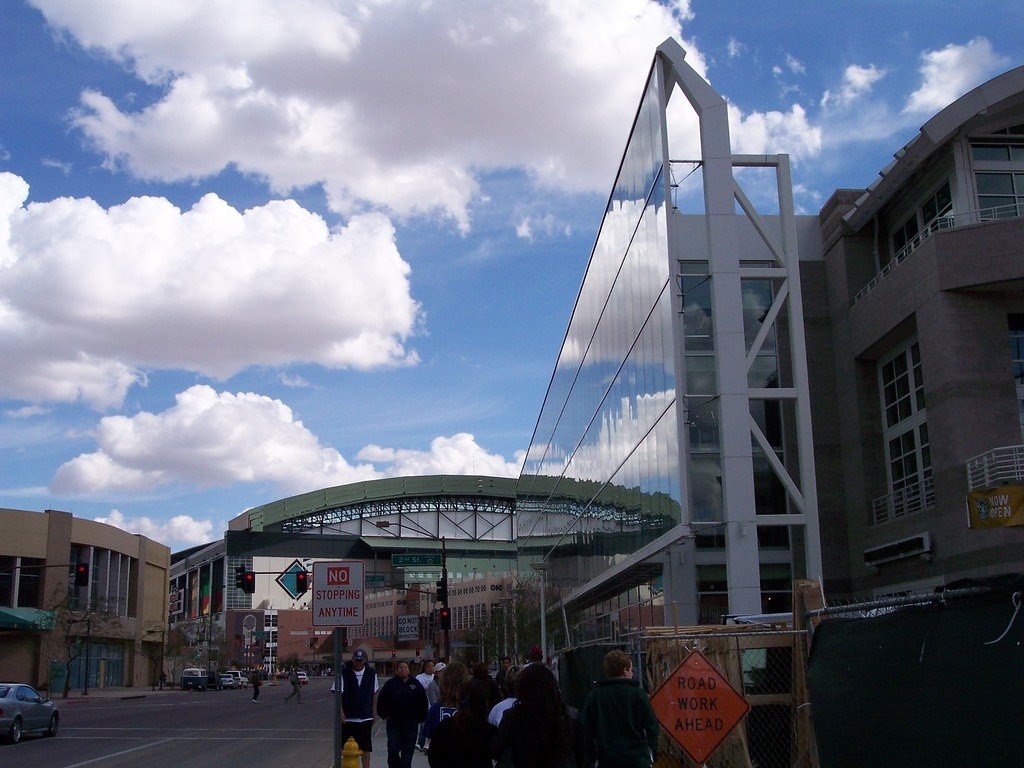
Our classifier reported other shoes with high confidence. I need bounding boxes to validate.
[424,744,429,751]
[415,744,421,751]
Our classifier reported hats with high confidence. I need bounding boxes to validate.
[434,662,446,672]
[352,649,367,660]
[530,645,542,656]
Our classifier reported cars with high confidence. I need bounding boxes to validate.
[179,667,248,692]
[277,671,289,679]
[296,671,308,685]
[0,682,61,744]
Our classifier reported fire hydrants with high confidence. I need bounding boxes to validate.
[341,735,364,768]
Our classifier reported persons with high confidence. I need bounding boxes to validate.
[331,643,660,768]
[252,670,259,703]
[286,667,303,703]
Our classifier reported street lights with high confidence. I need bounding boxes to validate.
[395,566,430,660]
[146,629,164,690]
[528,562,554,666]
[376,521,449,666]
[67,618,91,695]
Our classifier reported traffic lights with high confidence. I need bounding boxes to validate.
[75,562,89,586]
[235,564,245,588]
[436,578,445,602]
[296,570,307,592]
[244,572,256,593]
[440,607,451,630]
